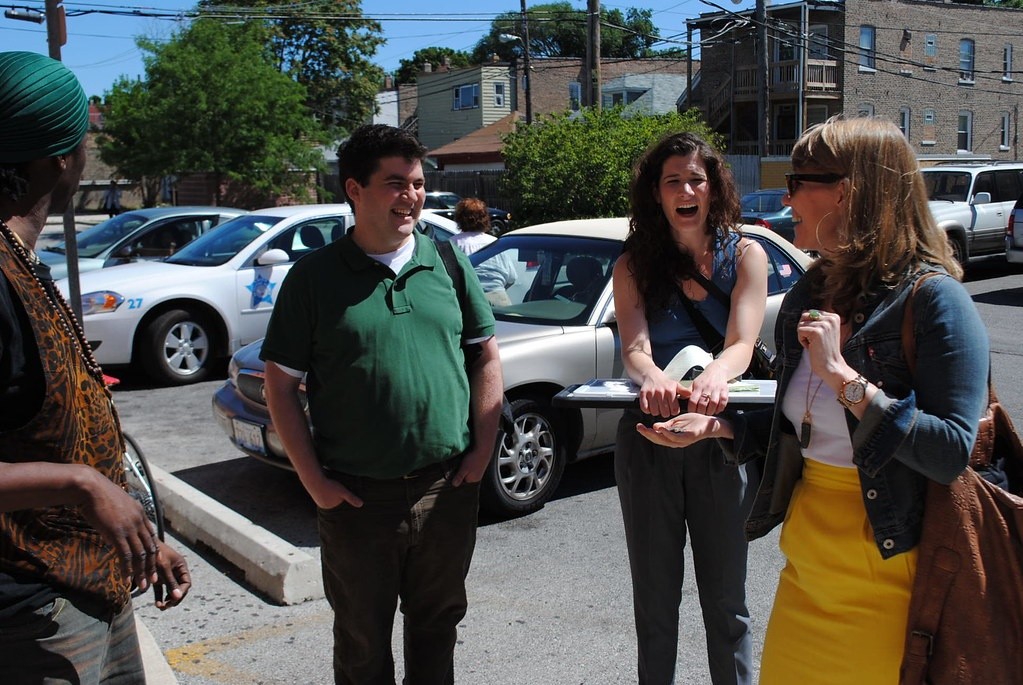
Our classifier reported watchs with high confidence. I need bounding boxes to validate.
[836,373,868,409]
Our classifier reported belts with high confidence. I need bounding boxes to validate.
[322,451,469,483]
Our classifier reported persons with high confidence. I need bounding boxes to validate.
[635,113,989,685]
[258,122,504,685]
[613,134,769,685]
[101,181,122,219]
[0,48,193,685]
[446,197,516,309]
[157,223,185,249]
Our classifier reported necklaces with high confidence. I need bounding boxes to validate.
[799,321,850,450]
[0,220,108,392]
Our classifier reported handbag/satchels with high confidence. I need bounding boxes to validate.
[886,271,1023,685]
[740,337,781,380]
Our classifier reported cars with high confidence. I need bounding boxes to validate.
[424,189,512,238]
[211,212,825,515]
[728,186,794,243]
[31,204,312,282]
[51,201,544,388]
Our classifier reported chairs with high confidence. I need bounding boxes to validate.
[552,258,607,303]
[298,227,326,254]
[328,223,342,244]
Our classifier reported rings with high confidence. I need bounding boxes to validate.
[702,392,711,400]
[809,311,819,321]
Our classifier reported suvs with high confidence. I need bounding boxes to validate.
[918,158,1023,281]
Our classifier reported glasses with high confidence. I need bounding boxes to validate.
[784,170,849,199]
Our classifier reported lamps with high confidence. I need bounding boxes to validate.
[903,28,912,40]
